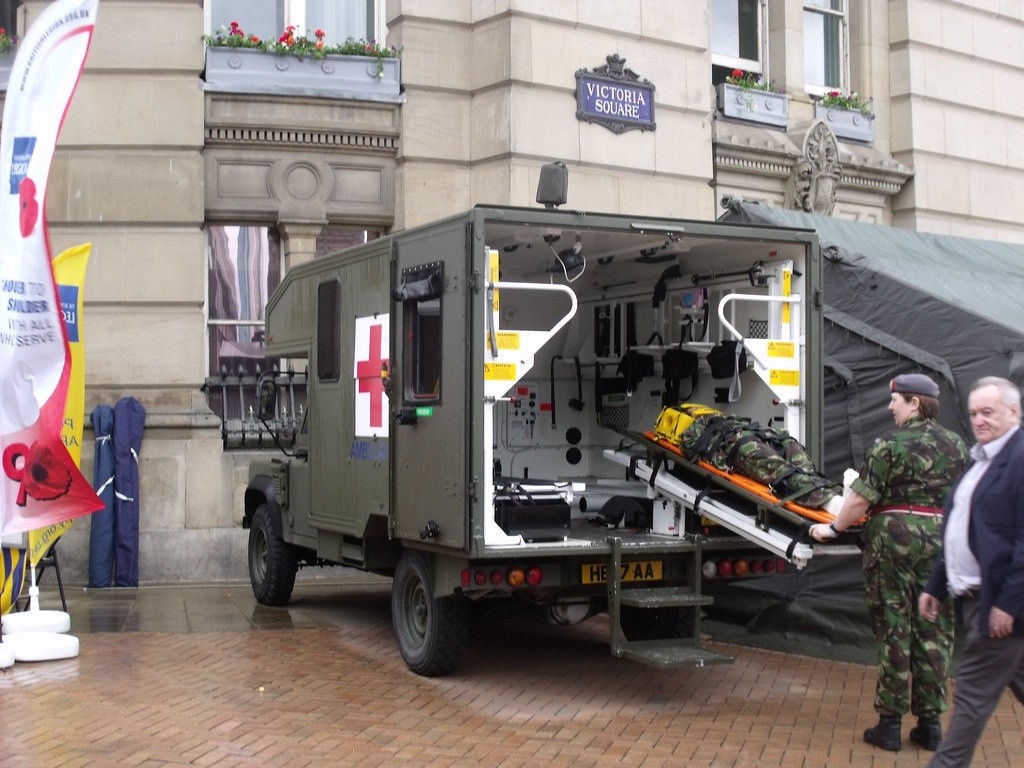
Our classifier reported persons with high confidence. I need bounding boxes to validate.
[918,377,1024,768]
[808,373,969,751]
[675,403,845,516]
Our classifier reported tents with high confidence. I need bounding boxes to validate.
[699,199,1024,667]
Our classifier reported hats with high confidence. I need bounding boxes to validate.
[889,373,940,398]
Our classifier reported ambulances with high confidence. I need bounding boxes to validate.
[243,162,827,677]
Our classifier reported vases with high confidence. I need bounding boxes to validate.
[814,100,874,142]
[717,82,790,128]
[205,44,400,95]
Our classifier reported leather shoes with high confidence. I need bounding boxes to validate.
[909,724,942,751]
[863,723,901,751]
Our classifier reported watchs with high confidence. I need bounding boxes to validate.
[829,520,839,533]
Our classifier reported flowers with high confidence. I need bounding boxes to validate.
[821,88,876,121]
[203,20,397,83]
[724,69,788,115]
